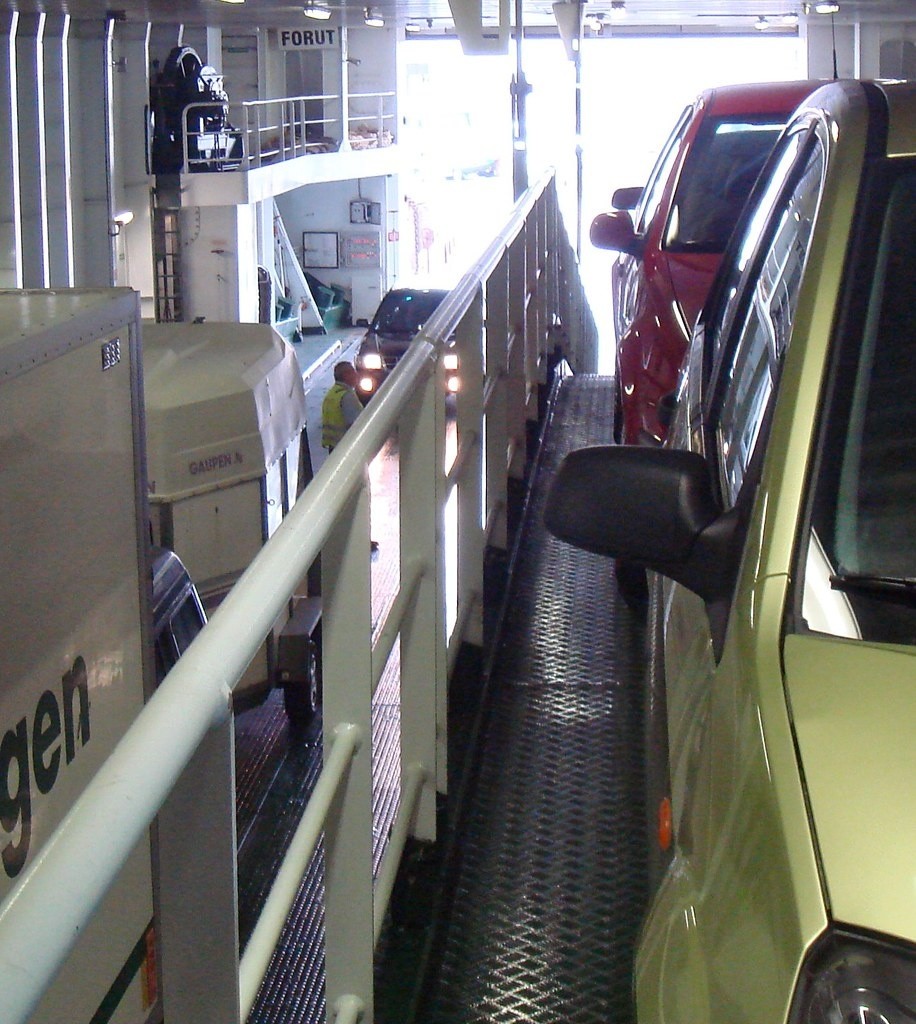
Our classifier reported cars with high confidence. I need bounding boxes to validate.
[355,288,489,406]
[545,78,915,1023]
[587,78,842,448]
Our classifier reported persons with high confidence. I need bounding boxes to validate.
[319,361,381,551]
